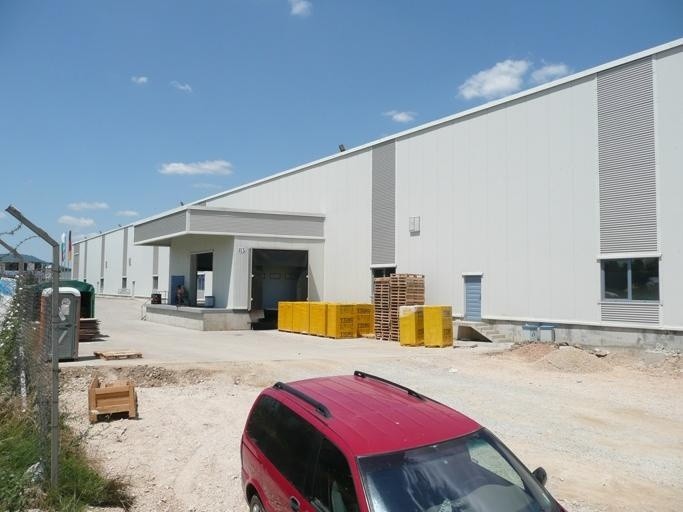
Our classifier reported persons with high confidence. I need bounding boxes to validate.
[176,284,185,306]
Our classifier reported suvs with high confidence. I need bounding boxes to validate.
[237,370,572,512]
